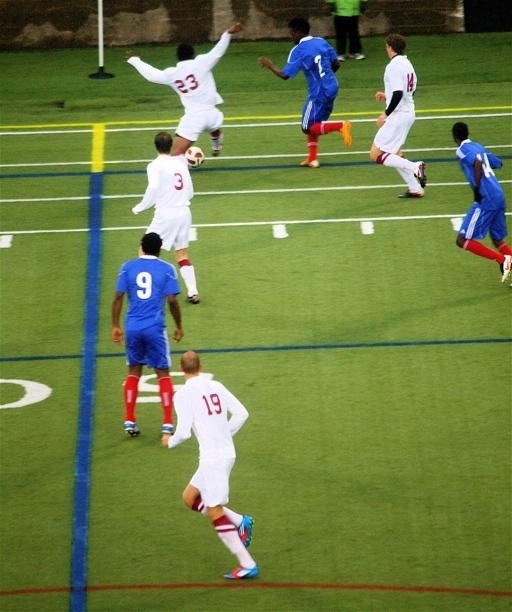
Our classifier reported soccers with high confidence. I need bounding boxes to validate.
[184,145,206,169]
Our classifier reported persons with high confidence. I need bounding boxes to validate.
[162,350,261,581]
[326,0,364,62]
[122,21,244,163]
[258,12,351,170]
[131,132,202,304]
[367,31,427,198]
[452,121,512,286]
[110,232,184,433]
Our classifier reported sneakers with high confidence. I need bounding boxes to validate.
[122,420,141,438]
[237,514,254,548]
[501,255,512,282]
[222,563,260,581]
[212,143,223,155]
[414,161,427,188]
[298,157,320,167]
[340,120,352,147]
[159,422,177,435]
[348,51,365,60]
[398,189,425,198]
[336,53,344,62]
[188,294,199,304]
